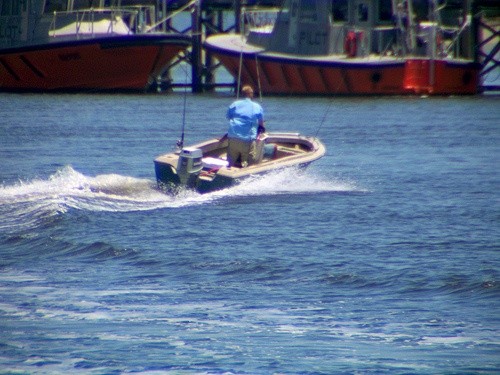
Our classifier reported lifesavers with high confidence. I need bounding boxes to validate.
[345,32,357,57]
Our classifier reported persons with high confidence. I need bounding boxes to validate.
[391,3,409,55]
[225,85,266,166]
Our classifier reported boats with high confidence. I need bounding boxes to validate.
[150,1,330,196]
[1,0,193,94]
[204,0,482,99]
